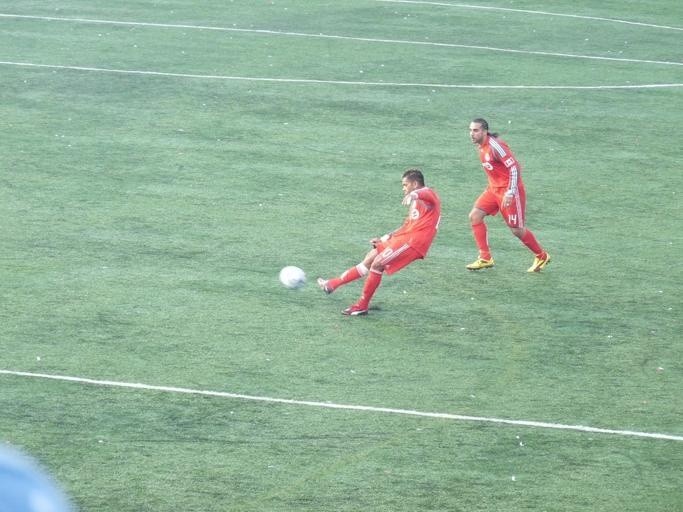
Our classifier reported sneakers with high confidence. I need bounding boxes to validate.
[527,252,551,273]
[466,256,494,270]
[318,278,334,294]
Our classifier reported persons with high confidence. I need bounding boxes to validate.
[317,170,441,316]
[465,118,551,273]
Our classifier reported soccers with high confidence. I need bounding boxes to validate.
[278,265,306,290]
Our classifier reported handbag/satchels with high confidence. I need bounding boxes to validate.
[341,306,368,315]
